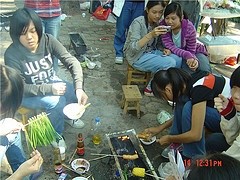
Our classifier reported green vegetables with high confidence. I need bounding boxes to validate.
[20,112,62,151]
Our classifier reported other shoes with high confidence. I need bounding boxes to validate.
[144,87,155,96]
[114,57,124,64]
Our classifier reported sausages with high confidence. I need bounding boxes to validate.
[122,153,138,160]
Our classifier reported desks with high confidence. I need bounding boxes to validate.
[201,9,240,38]
[206,45,240,78]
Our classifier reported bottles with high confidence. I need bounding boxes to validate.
[77,133,85,156]
[92,117,102,145]
[53,146,62,174]
[58,138,65,161]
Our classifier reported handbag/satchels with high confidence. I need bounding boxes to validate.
[92,5,112,20]
[158,149,185,180]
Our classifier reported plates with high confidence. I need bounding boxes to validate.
[72,176,89,180]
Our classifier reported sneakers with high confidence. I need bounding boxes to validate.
[63,116,84,129]
[160,142,184,159]
[51,135,68,151]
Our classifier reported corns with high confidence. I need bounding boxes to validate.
[132,167,145,177]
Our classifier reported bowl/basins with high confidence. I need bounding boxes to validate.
[140,136,156,145]
[71,158,90,173]
[62,103,86,120]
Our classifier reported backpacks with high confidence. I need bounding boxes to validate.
[89,0,101,14]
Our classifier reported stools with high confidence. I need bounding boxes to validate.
[121,84,143,118]
[126,63,148,86]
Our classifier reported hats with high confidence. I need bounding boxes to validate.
[231,66,240,88]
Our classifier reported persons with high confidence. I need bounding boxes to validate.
[0,0,88,180]
[102,0,240,180]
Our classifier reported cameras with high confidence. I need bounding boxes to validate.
[159,26,171,33]
